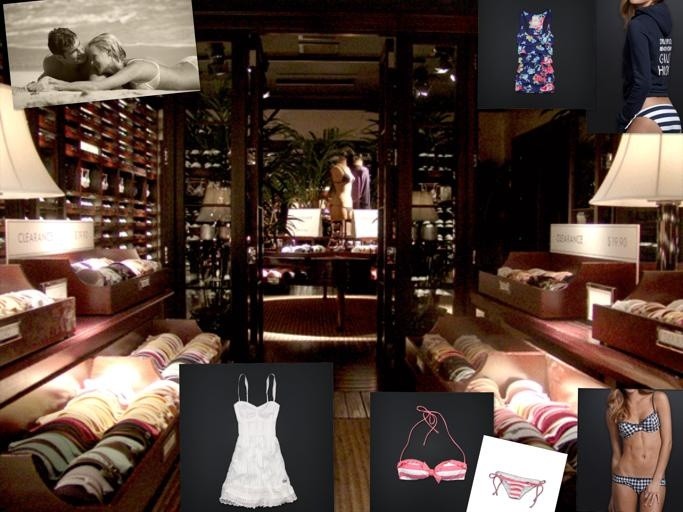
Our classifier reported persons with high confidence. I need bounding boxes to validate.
[330,155,355,223]
[616,1,682,135]
[347,154,374,208]
[23,24,121,91]
[599,388,673,511]
[37,32,200,91]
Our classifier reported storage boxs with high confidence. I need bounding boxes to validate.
[7,247,169,314]
[0,355,179,512]
[94,319,230,380]
[403,314,543,391]
[462,353,612,488]
[593,272,682,376]
[0,264,75,369]
[478,252,637,317]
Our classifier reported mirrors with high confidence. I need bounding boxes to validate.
[396,38,465,362]
[159,36,256,358]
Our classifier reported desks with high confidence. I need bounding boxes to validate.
[465,285,681,395]
[261,246,379,332]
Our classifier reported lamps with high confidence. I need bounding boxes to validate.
[428,51,451,74]
[0,84,66,200]
[208,43,227,77]
[194,187,230,282]
[411,191,438,245]
[414,77,433,97]
[589,133,682,270]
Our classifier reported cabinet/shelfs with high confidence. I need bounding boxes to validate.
[0,98,161,256]
[0,291,231,511]
